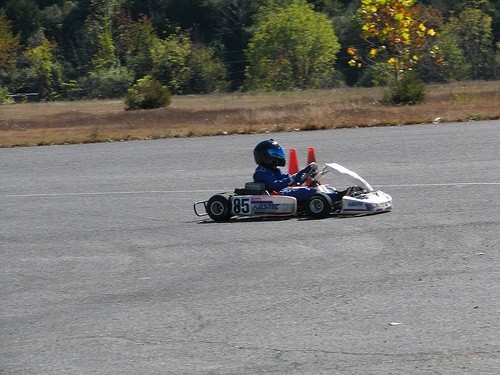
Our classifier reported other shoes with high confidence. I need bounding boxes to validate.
[339,186,355,201]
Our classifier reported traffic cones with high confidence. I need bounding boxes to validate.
[306,147,316,164]
[287,149,298,175]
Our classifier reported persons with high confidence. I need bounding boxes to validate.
[252,138,319,202]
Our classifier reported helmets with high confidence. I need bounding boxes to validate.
[253,139,287,167]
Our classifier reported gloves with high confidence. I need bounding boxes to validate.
[296,162,318,177]
